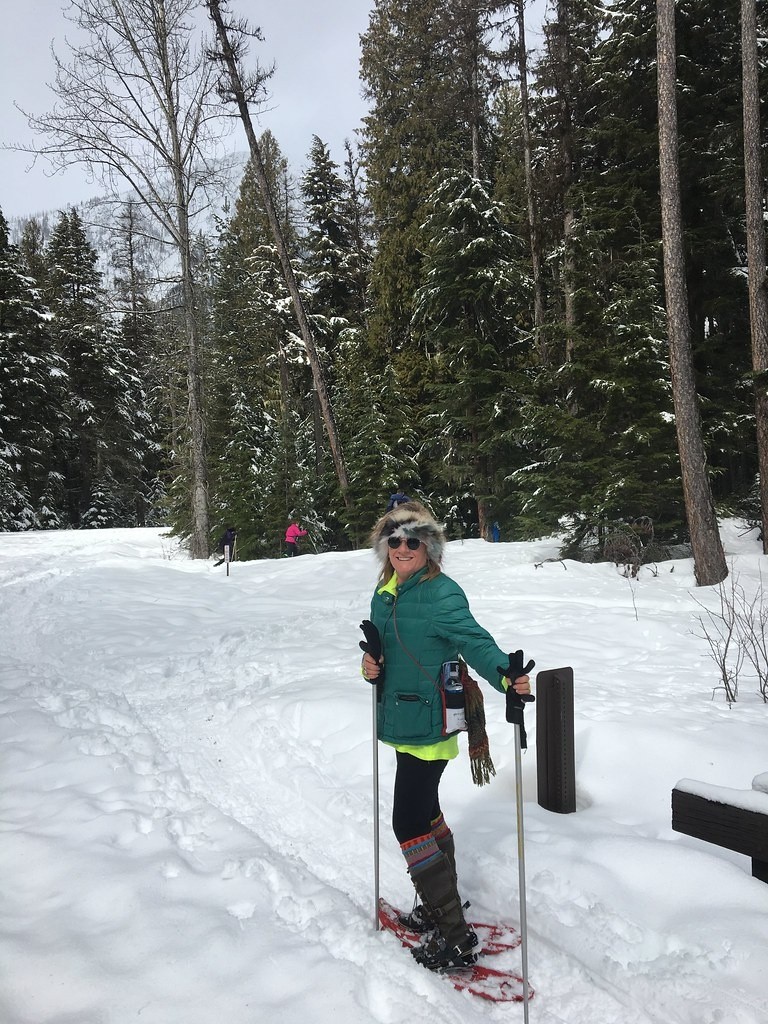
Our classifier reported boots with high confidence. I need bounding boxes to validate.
[397,829,453,933]
[410,854,478,971]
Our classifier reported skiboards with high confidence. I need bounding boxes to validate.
[378,897,534,1002]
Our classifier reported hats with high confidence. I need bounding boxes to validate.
[371,503,445,562]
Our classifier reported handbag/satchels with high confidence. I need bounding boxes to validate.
[439,682,469,736]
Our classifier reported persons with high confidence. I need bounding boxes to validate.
[212,526,239,567]
[283,519,308,558]
[491,522,501,542]
[359,497,533,973]
[386,489,412,513]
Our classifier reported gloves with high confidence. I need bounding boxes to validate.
[358,620,385,682]
[496,650,535,724]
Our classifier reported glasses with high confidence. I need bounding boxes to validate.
[386,538,421,551]
